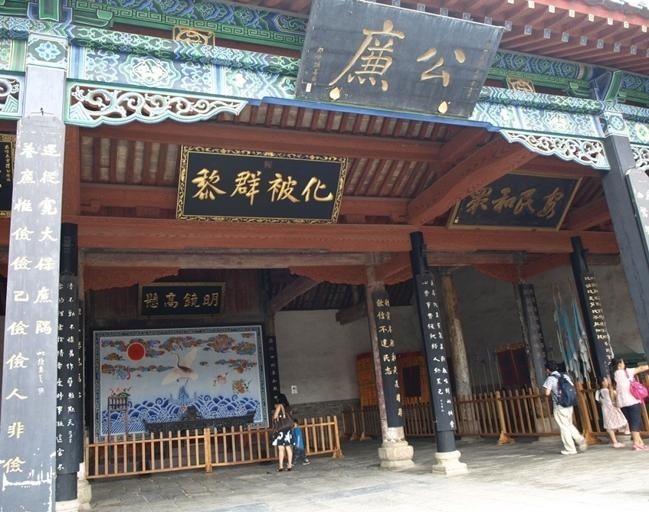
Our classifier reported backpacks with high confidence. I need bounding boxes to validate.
[550,373,577,407]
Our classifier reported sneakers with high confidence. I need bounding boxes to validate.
[561,450,577,455]
[302,460,311,465]
[286,463,294,467]
[579,438,587,451]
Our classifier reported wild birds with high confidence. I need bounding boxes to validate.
[160,346,200,392]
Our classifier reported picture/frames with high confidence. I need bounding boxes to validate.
[90,323,272,448]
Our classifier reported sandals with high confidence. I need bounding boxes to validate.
[279,468,292,471]
[613,443,625,448]
[633,443,649,451]
[625,431,630,434]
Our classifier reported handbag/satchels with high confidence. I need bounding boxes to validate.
[623,369,649,399]
[277,418,291,431]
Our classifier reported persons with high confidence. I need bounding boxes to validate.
[271,393,311,472]
[595,357,649,451]
[542,360,587,456]
